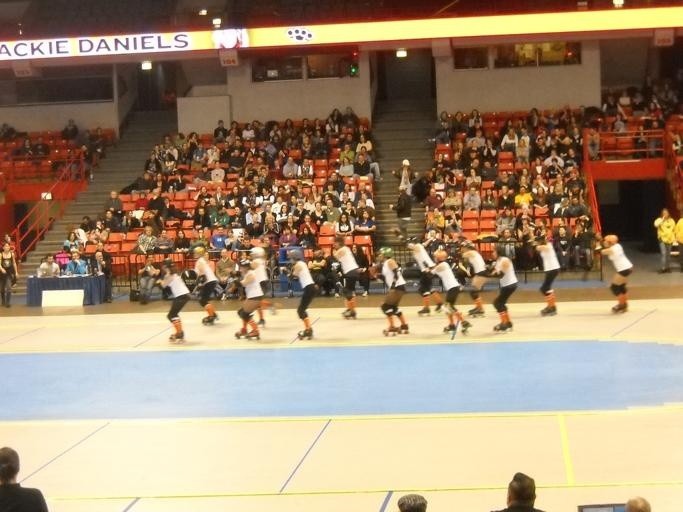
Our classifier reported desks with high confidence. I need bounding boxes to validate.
[27,275,112,307]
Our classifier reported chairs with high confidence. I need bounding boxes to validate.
[0,127,116,182]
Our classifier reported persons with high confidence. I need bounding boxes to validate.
[398,494,427,512]
[0,73,683,307]
[625,496,651,512]
[376,248,410,337]
[534,237,560,316]
[490,472,546,512]
[1,447,48,512]
[153,258,191,341]
[191,245,220,326]
[231,248,270,341]
[280,250,318,340]
[330,237,359,320]
[597,234,632,314]
[410,238,518,333]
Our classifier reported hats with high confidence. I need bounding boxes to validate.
[403,160,409,166]
